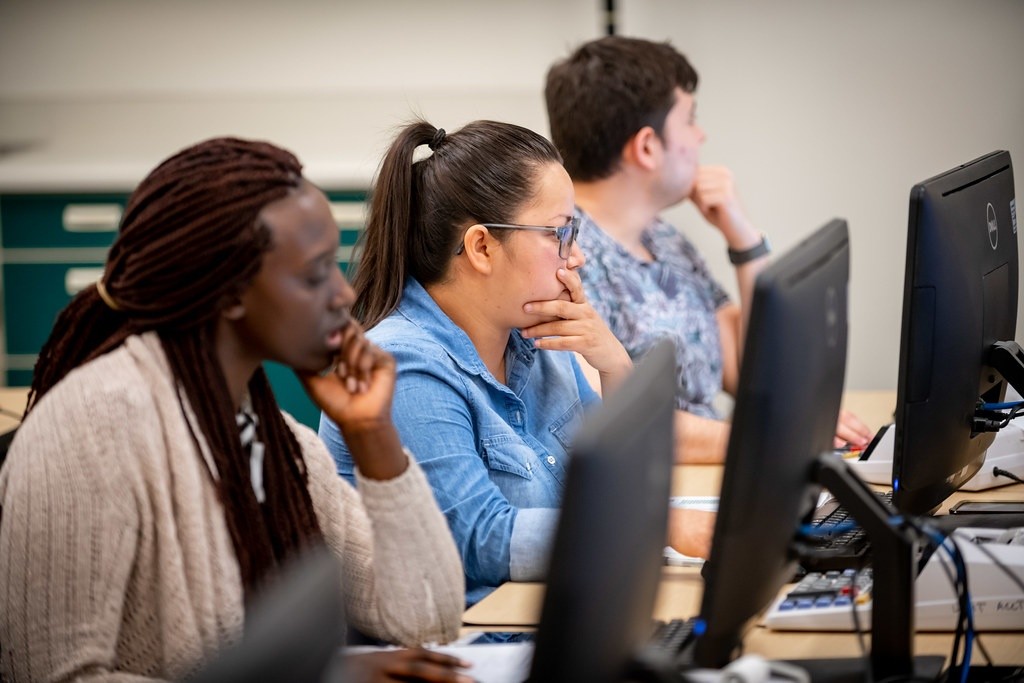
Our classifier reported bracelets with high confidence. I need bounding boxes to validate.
[726,238,769,263]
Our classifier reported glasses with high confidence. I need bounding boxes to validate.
[454,216,582,260]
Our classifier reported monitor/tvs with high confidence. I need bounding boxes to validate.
[526,341,688,683]
[689,218,947,683]
[180,553,351,683]
[889,149,1024,515]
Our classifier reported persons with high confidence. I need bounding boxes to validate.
[319,122,718,646]
[545,38,872,467]
[0,137,476,683]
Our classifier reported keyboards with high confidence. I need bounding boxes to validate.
[649,616,701,670]
[794,491,899,572]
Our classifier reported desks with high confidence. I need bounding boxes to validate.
[459,388,1024,683]
